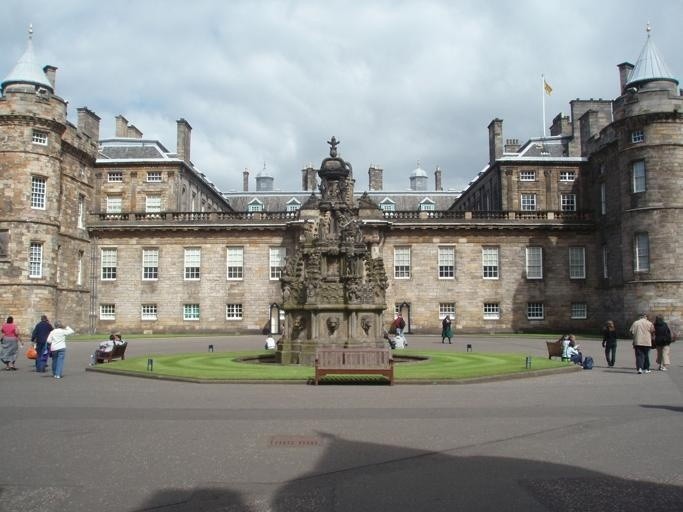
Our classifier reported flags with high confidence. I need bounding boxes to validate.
[544,80,553,96]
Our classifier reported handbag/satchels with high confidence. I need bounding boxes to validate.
[26,348,37,359]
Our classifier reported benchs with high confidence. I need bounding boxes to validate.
[93,340,127,364]
[310,346,394,386]
[545,339,563,360]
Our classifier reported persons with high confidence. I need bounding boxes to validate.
[441,315,453,344]
[0,315,23,370]
[46,319,75,379]
[264,333,276,349]
[395,314,408,346]
[629,312,656,374]
[31,315,54,372]
[115,334,125,345]
[654,314,672,371]
[88,335,116,366]
[556,334,583,366]
[388,320,397,334]
[602,320,617,366]
